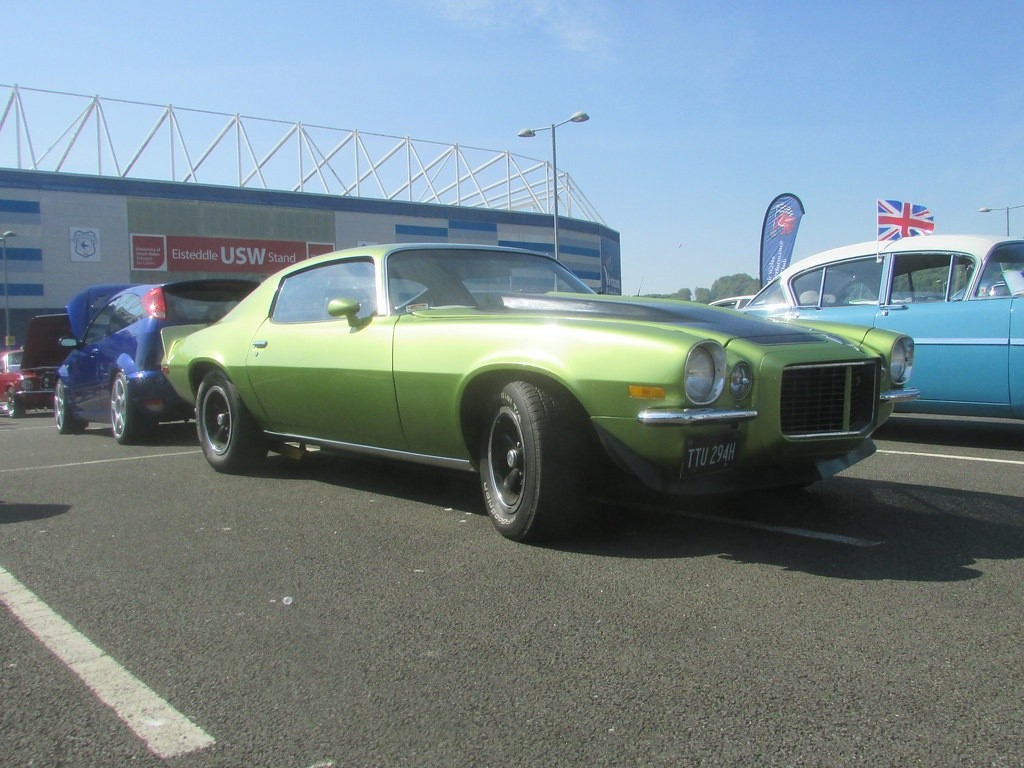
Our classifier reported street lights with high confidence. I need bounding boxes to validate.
[978,204,1024,237]
[518,112,589,295]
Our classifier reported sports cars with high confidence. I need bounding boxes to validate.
[157,244,916,542]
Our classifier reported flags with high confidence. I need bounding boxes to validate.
[759,192,805,291]
[876,198,940,242]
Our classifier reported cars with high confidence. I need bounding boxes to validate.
[53,279,262,445]
[707,295,755,312]
[0,313,76,418]
[739,237,1024,420]
